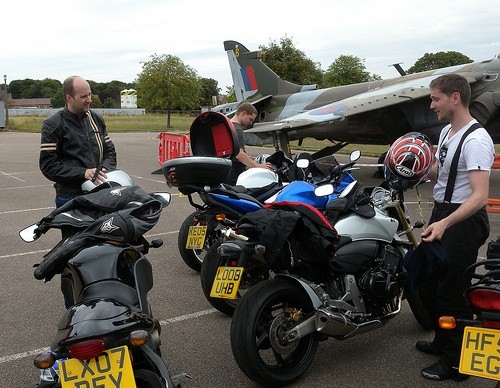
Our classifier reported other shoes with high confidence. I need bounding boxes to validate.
[416,340,444,356]
[421,360,457,380]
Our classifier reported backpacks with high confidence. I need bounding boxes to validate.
[263,201,340,244]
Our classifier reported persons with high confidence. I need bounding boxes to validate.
[405,72,496,379]
[221,101,271,186]
[39,74,117,309]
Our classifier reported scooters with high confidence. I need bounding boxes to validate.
[438,237,500,388]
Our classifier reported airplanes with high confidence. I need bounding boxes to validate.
[203,40,500,152]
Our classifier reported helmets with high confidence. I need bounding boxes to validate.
[81,169,133,194]
[384,132,434,193]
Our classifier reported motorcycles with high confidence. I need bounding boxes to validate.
[151,110,350,272]
[18,170,182,388]
[184,150,366,313]
[213,163,427,388]
